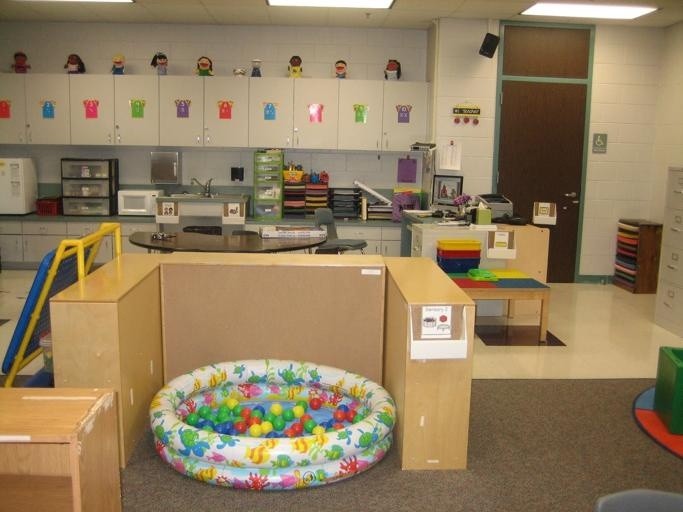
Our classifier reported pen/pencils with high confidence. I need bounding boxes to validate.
[417,213,442,217]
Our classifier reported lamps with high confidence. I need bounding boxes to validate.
[520,1,658,22]
[269,0,394,11]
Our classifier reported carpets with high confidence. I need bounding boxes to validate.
[118,376,683,512]
[631,376,683,461]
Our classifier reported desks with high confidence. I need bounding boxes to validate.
[446,269,551,348]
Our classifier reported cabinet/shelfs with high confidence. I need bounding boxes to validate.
[0,385,118,512]
[68,222,112,274]
[0,72,68,145]
[654,166,683,340]
[339,78,428,152]
[0,217,22,268]
[406,210,549,318]
[22,220,66,271]
[249,75,339,149]
[247,226,402,254]
[70,73,158,145]
[113,218,162,256]
[613,218,664,294]
[160,73,249,146]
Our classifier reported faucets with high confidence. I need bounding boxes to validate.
[192,177,213,198]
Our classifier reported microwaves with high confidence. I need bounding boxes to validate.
[118,189,165,216]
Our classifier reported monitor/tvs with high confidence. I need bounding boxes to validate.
[431,174,464,218]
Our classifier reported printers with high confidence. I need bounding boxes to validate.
[478,194,512,220]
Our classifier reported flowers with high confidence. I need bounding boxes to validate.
[452,193,470,210]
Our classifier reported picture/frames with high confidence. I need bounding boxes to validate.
[432,174,466,206]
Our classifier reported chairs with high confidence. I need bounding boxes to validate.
[312,204,366,254]
